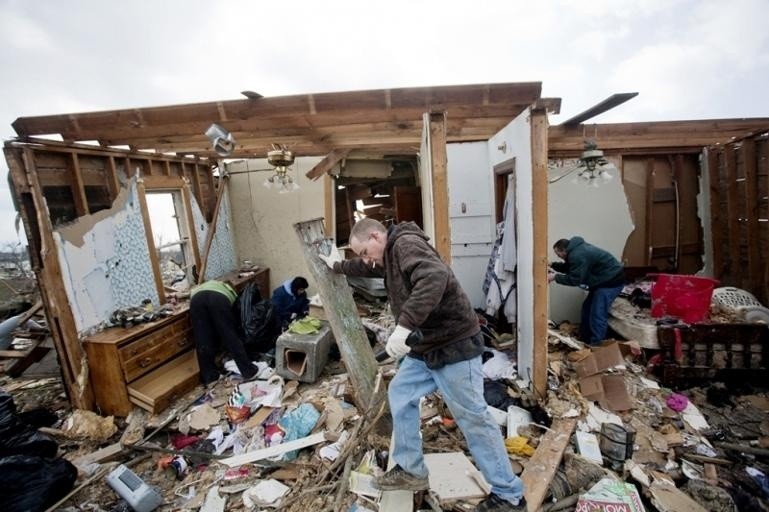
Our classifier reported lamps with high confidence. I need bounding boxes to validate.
[261,142,301,196]
[575,138,618,190]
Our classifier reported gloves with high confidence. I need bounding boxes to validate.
[318,243,342,270]
[385,323,412,360]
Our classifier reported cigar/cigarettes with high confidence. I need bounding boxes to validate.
[373,261,376,268]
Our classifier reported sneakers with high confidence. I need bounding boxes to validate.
[370,464,430,491]
[248,363,265,381]
[472,491,528,512]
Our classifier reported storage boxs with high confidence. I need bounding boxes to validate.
[104,461,164,511]
[646,270,721,326]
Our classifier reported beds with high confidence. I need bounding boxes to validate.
[599,264,768,395]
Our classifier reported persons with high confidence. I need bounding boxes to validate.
[549,236,620,345]
[320,217,528,511]
[254,278,312,352]
[189,274,258,387]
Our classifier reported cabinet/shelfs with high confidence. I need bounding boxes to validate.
[82,296,202,422]
[214,265,271,300]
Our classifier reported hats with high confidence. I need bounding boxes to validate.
[290,277,309,294]
